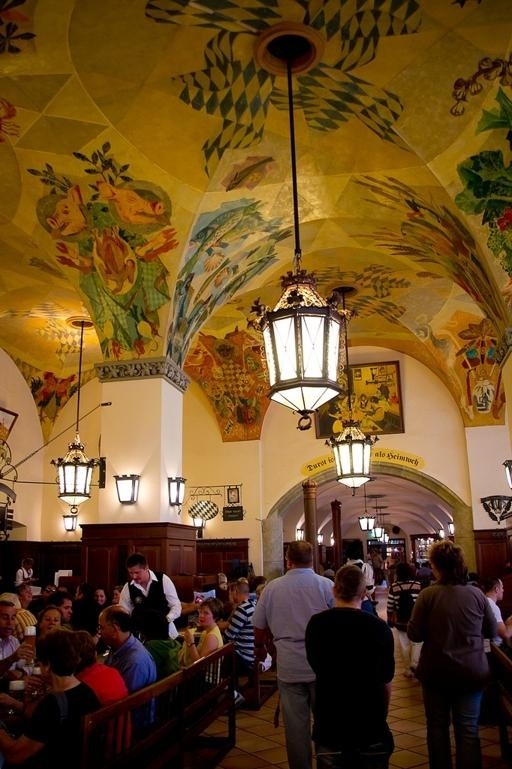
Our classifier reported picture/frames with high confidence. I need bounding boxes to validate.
[227,488,239,503]
[313,360,405,439]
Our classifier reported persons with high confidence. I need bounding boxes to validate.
[406,538,497,769]
[305,564,396,769]
[93,554,183,648]
[2,577,268,768]
[14,557,40,600]
[251,541,335,769]
[323,539,512,704]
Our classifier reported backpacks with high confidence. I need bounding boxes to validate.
[396,581,415,623]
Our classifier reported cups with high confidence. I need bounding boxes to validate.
[188,615,196,630]
[32,667,40,680]
[24,626,36,659]
[9,681,25,703]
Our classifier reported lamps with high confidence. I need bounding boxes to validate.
[248,59,355,430]
[297,529,304,541]
[113,475,141,503]
[438,529,444,539]
[447,523,454,535]
[502,459,512,489]
[358,483,390,545]
[324,294,380,499]
[168,476,187,507]
[318,534,323,543]
[193,519,207,528]
[63,515,77,531]
[50,321,101,514]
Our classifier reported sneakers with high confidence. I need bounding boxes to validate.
[223,693,245,715]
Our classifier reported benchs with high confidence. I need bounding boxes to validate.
[83,642,238,769]
[231,644,278,704]
[490,639,512,769]
